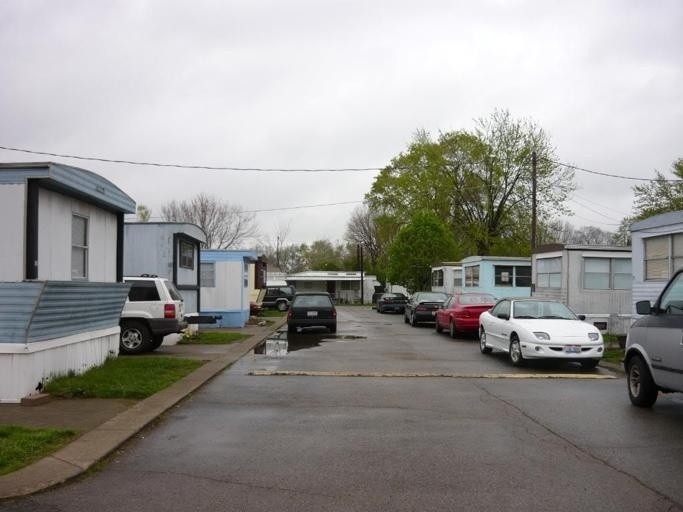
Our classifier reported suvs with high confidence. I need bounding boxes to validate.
[119,272,194,355]
[259,282,298,312]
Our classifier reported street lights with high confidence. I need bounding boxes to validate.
[359,239,365,306]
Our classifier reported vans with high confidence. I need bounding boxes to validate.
[622,266,683,413]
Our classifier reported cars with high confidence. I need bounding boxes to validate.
[284,290,342,336]
[374,290,409,315]
[473,296,610,367]
[428,291,503,339]
[401,291,452,329]
